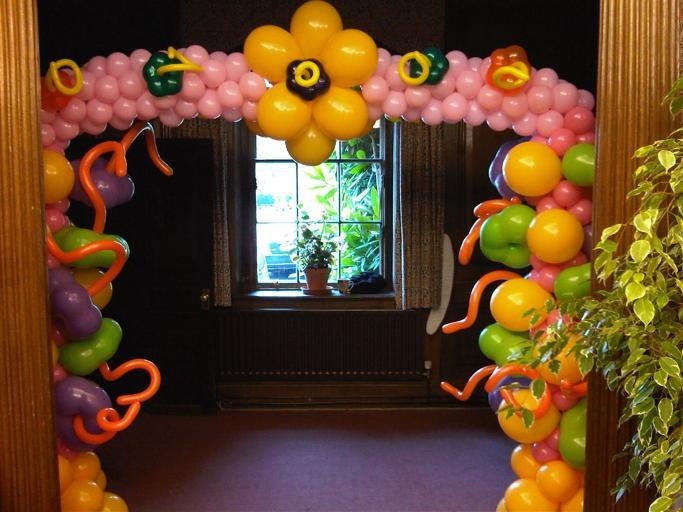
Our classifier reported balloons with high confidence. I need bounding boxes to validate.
[38,45,268,153]
[438,138,596,511]
[361,44,597,154]
[44,121,175,512]
[243,1,378,165]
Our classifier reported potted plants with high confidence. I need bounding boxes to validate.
[291,203,340,290]
